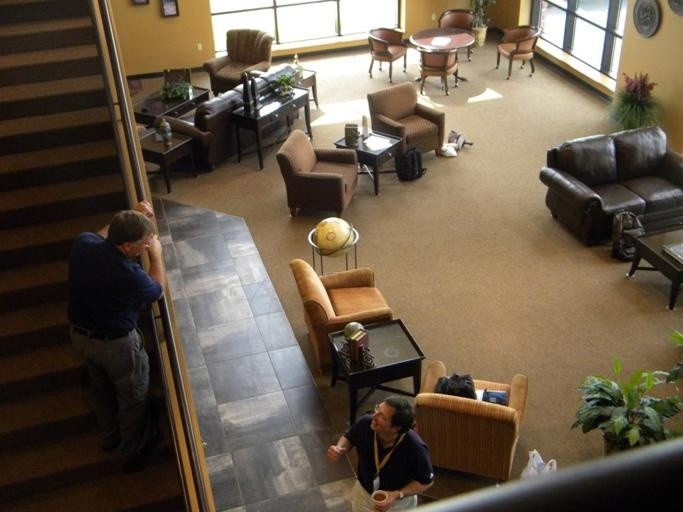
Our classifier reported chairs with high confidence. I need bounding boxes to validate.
[203,28,276,99]
[496,25,540,81]
[413,359,530,488]
[367,82,445,166]
[277,129,357,218]
[439,9,475,61]
[286,256,392,376]
[368,27,407,83]
[418,47,459,96]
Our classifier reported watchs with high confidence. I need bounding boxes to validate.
[399,490,405,501]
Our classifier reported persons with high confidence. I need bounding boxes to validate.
[63,204,167,470]
[325,397,435,511]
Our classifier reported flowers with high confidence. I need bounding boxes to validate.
[611,70,664,127]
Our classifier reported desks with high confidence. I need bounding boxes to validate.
[336,129,405,194]
[232,85,313,168]
[130,86,211,129]
[324,316,425,427]
[408,27,476,82]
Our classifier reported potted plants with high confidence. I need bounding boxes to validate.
[567,352,681,457]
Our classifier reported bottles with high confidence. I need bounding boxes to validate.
[291,52,302,78]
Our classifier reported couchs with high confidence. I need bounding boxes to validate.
[159,64,298,171]
[540,124,681,247]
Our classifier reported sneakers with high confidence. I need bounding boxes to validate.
[101,425,161,458]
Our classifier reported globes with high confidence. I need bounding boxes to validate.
[307,218,359,275]
[343,321,364,342]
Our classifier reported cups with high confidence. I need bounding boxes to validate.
[370,489,389,507]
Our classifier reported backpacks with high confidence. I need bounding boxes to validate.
[612,207,646,262]
[395,143,426,182]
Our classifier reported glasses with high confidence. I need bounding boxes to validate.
[374,405,397,428]
